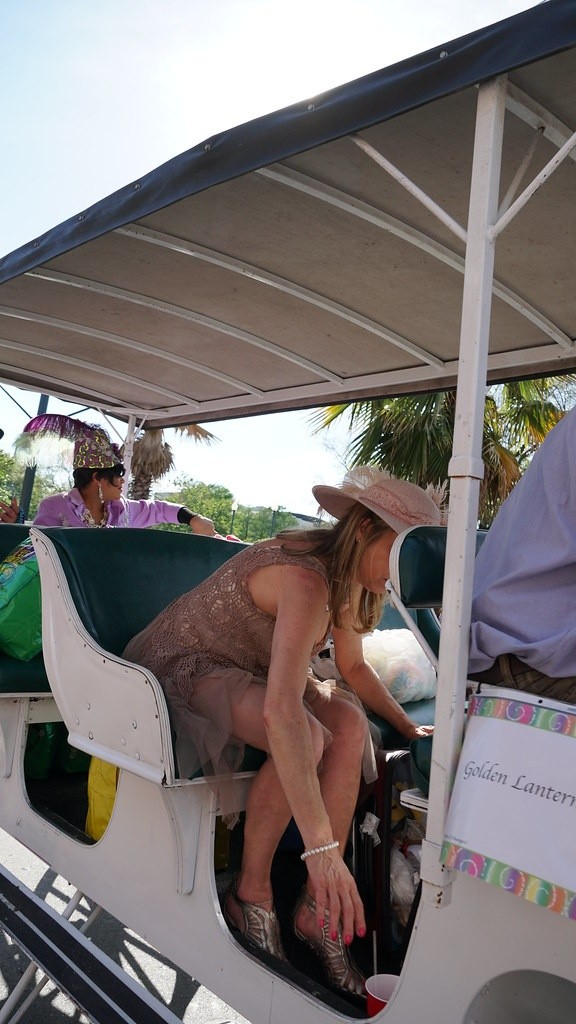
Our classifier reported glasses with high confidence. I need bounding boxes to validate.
[105,470,126,477]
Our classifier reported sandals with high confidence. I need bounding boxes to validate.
[294,894,367,1000]
[223,880,291,970]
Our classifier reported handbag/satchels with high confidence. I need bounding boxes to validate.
[0,536,42,663]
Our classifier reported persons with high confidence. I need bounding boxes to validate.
[123,467,448,999]
[468,405,575,706]
[0,429,26,524]
[14,413,222,536]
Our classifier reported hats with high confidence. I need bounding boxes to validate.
[312,466,448,535]
[12,414,123,468]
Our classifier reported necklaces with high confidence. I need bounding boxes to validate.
[5,506,25,524]
[82,504,108,528]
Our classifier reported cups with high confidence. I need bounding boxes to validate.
[365,973,400,1017]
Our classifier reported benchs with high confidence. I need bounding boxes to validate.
[0,522,576,923]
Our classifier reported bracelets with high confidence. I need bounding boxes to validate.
[301,842,339,861]
